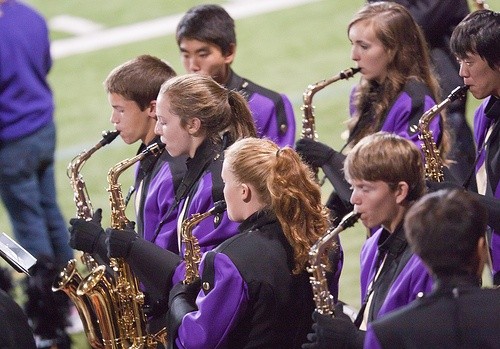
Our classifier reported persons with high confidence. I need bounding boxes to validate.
[368,0,479,203]
[168,136,345,349]
[67,52,181,338]
[174,2,298,154]
[0,0,500,349]
[297,0,449,234]
[0,264,39,349]
[1,0,76,337]
[303,129,440,349]
[450,9,500,288]
[103,71,258,310]
[362,188,500,349]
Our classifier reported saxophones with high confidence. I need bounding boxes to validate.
[75,142,159,349]
[417,85,470,183]
[151,200,226,349]
[306,211,360,314]
[300,64,361,195]
[51,127,119,348]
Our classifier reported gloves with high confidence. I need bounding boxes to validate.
[105,221,186,304]
[301,302,365,349]
[68,208,120,289]
[166,278,202,349]
[295,138,353,209]
[431,182,500,233]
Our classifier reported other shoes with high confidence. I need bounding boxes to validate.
[64,306,84,334]
